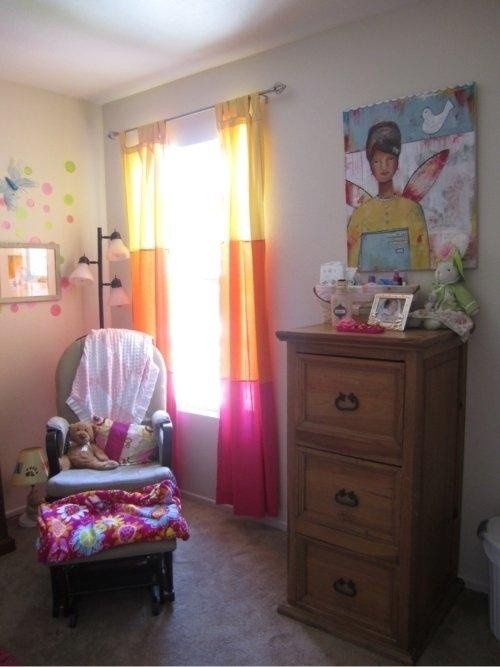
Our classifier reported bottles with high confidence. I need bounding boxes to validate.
[368,270,407,286]
[330,278,353,325]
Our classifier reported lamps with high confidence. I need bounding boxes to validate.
[69,227,130,328]
[12,447,49,528]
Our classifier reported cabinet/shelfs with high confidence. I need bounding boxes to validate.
[276,324,467,666]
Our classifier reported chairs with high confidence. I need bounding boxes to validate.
[45,325,174,502]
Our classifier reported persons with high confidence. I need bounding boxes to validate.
[378,298,401,324]
[346,119,450,270]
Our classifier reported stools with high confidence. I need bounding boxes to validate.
[50,538,175,628]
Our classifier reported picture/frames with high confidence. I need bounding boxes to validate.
[368,292,413,331]
[0,243,61,302]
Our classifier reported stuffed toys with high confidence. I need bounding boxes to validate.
[56,419,121,471]
[406,244,479,343]
